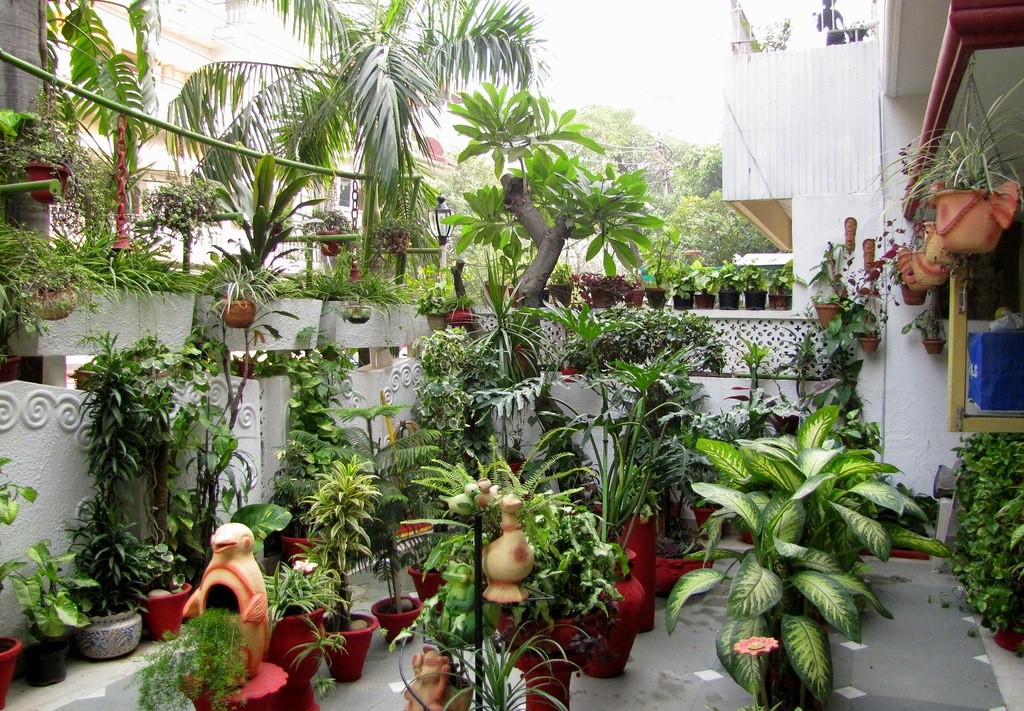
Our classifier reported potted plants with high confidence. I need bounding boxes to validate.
[0,82,1024,711]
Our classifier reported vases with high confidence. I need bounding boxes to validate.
[264,605,322,711]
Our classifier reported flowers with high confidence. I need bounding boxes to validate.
[260,557,352,698]
